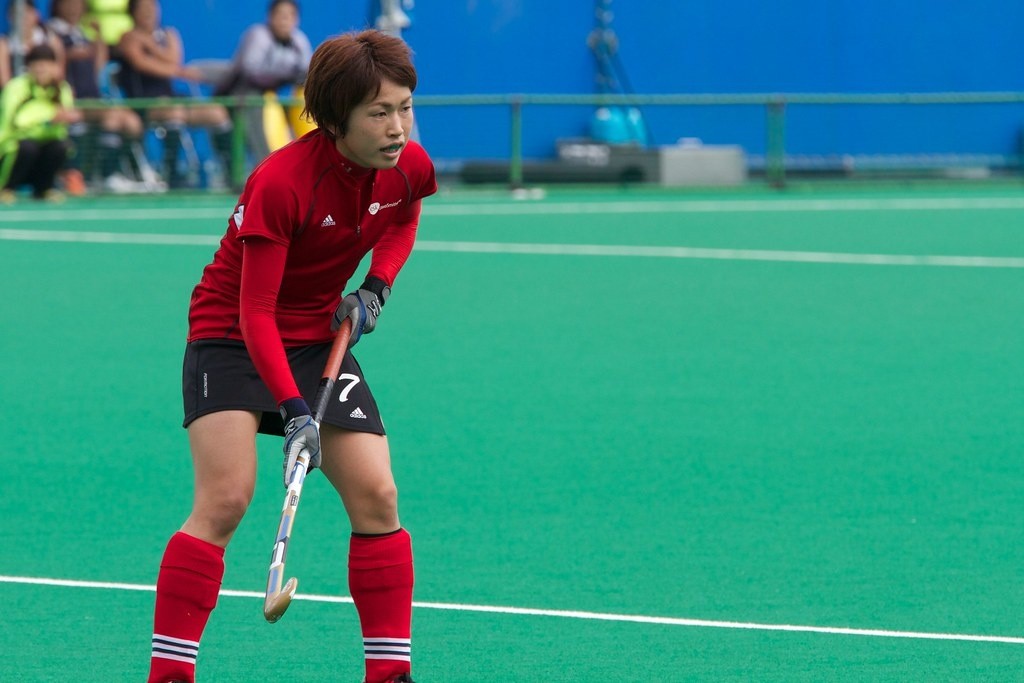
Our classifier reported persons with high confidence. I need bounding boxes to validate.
[144,30,439,683]
[0,1,312,206]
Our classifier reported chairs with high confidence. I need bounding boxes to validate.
[107,60,236,191]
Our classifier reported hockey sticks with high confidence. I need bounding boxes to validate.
[261,314,354,627]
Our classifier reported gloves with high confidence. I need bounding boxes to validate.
[279,400,323,488]
[331,275,390,351]
